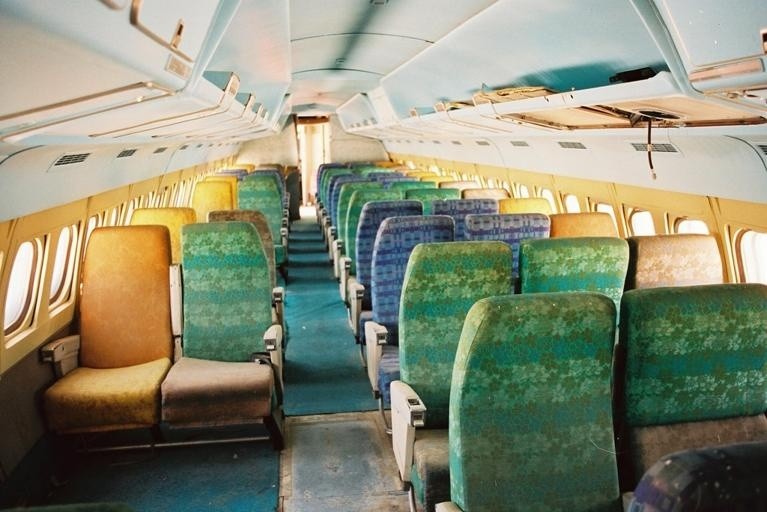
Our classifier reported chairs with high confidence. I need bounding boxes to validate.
[314,161,767,511]
[38,163,291,455]
